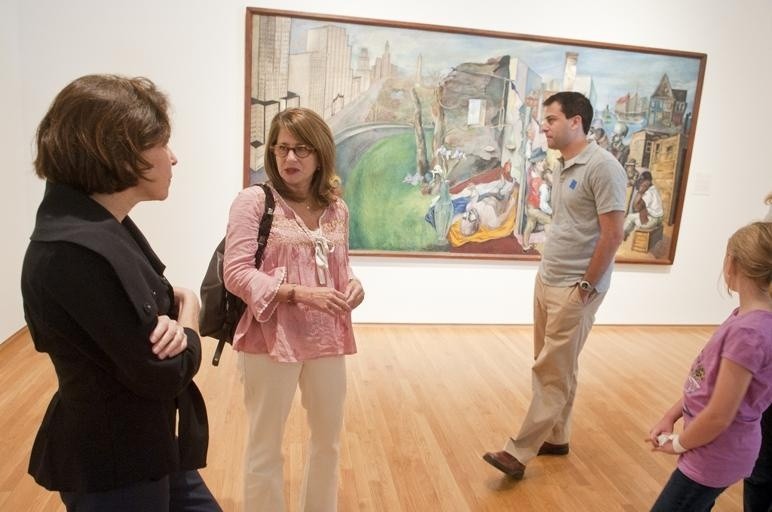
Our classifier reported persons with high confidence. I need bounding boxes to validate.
[482,92,629,481]
[221,106,366,512]
[19,72,224,512]
[641,222,772,512]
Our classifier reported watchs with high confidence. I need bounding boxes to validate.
[579,279,592,292]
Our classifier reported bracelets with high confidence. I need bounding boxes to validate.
[671,432,687,455]
[286,282,298,305]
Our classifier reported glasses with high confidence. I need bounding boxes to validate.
[268,142,315,159]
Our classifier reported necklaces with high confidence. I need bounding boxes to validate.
[306,205,311,210]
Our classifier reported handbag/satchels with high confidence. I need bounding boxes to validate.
[198,184,277,368]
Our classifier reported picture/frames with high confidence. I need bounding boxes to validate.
[244,6,709,267]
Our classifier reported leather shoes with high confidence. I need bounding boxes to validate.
[538,442,570,455]
[482,449,526,481]
